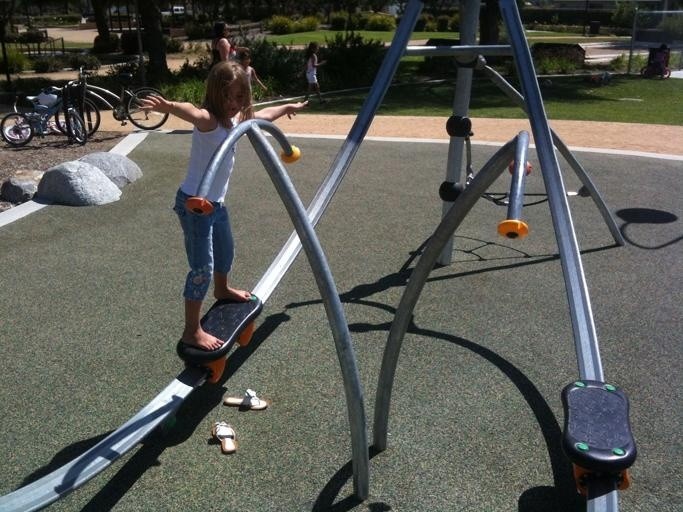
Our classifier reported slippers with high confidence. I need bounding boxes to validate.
[211,419,238,456]
[223,388,267,410]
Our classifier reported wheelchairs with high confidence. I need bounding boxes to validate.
[640,46,670,79]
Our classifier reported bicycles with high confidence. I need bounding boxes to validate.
[55,64,170,137]
[5,116,74,139]
[1,79,88,147]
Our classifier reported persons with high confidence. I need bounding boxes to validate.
[236,50,266,120]
[209,21,250,66]
[295,41,330,106]
[135,61,316,353]
